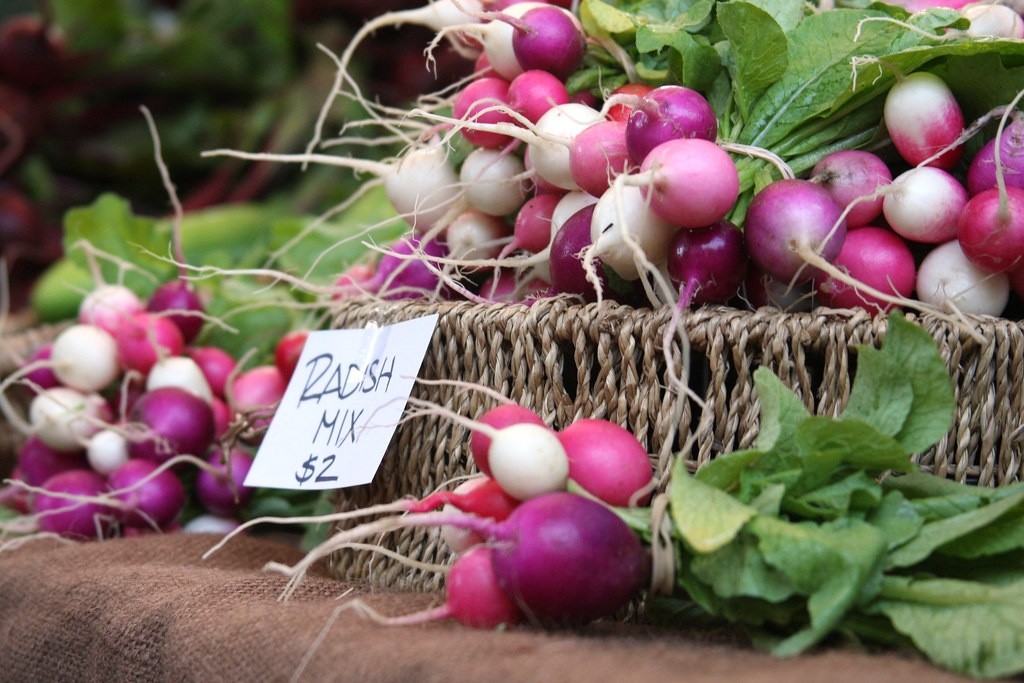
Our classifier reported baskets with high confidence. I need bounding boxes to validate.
[293,298,1024,588]
[0,317,111,455]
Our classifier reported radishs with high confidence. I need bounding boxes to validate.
[18,1,1008,549]
